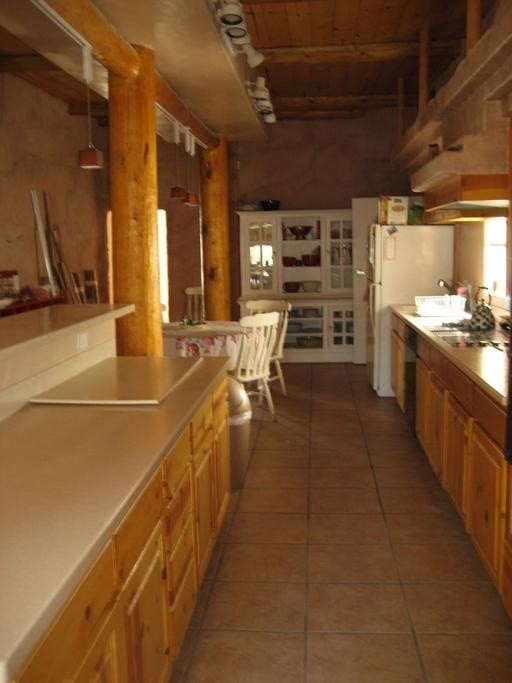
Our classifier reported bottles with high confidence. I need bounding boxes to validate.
[408,200,416,225]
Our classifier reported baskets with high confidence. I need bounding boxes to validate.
[413,295,467,316]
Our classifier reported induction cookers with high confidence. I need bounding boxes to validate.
[433,328,511,349]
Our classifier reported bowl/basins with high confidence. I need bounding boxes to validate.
[285,322,322,348]
[263,200,281,208]
[286,225,313,240]
[284,254,317,266]
[284,280,320,292]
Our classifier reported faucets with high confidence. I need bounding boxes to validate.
[437,279,451,292]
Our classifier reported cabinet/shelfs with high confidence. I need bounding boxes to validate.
[189,391,217,593]
[235,209,354,364]
[415,359,446,487]
[443,392,512,618]
[15,537,132,683]
[161,422,197,664]
[211,374,232,544]
[389,313,407,413]
[113,466,172,683]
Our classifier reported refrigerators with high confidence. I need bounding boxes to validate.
[368,224,454,398]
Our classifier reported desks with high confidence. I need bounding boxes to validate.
[163,318,257,389]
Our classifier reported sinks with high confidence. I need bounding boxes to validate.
[403,305,417,311]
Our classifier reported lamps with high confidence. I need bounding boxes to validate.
[166,121,199,210]
[78,85,103,170]
[214,0,278,127]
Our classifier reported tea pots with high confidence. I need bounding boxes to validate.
[472,286,495,329]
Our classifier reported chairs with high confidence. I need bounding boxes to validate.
[185,285,287,422]
